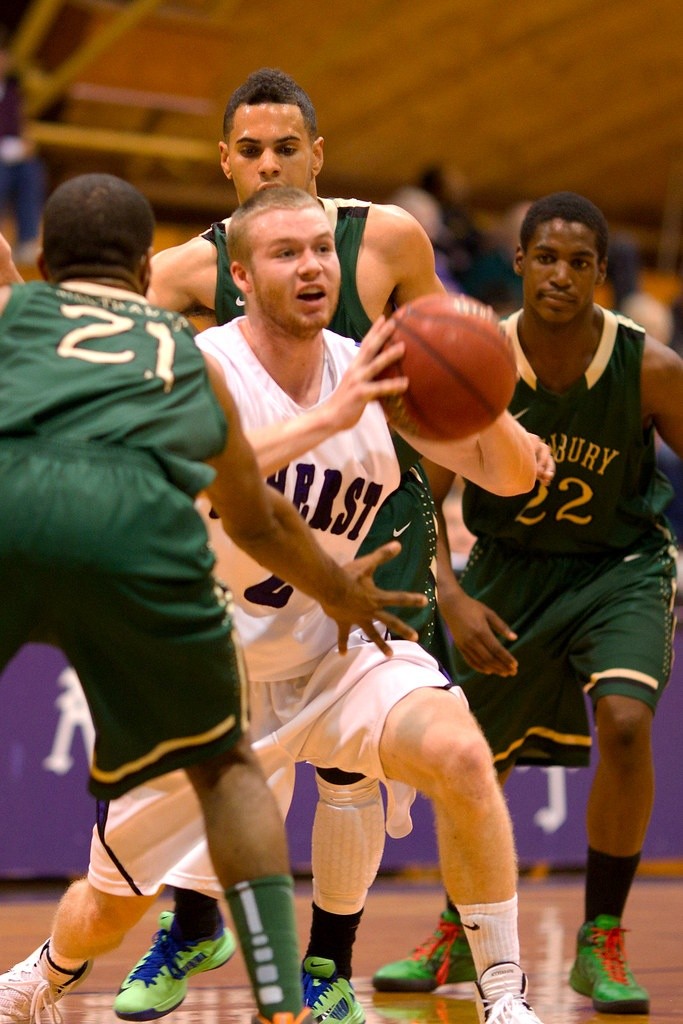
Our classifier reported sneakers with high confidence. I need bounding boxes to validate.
[0,937,94,1024]
[372,909,477,991]
[251,1006,314,1024]
[113,910,237,1022]
[568,914,649,1013]
[472,962,543,1024]
[299,957,366,1024]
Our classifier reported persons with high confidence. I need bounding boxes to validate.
[0,66,683,1024]
[0,184,547,1024]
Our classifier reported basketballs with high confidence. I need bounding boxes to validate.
[368,294,517,441]
